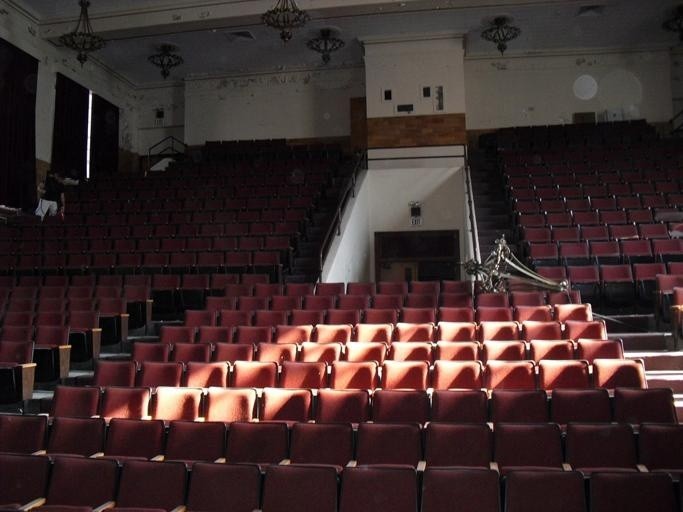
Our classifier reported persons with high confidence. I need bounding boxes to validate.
[35,172,66,222]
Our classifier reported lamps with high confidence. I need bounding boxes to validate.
[147,44,185,79]
[261,0,311,47]
[307,29,346,63]
[63,1,106,71]
[481,17,521,56]
